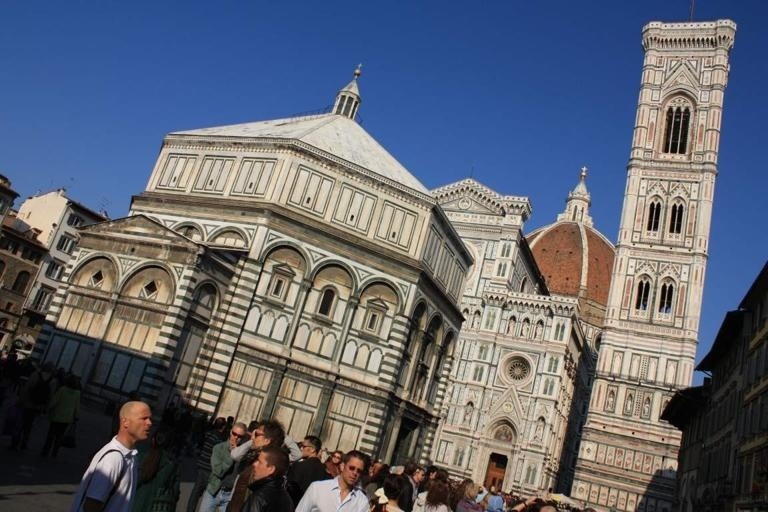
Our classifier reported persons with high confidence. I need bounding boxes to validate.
[153,395,414,512]
[70,401,152,512]
[2,344,81,462]
[111,391,141,439]
[407,464,597,512]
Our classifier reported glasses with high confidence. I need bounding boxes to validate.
[229,429,244,439]
[255,431,265,438]
[303,444,311,449]
